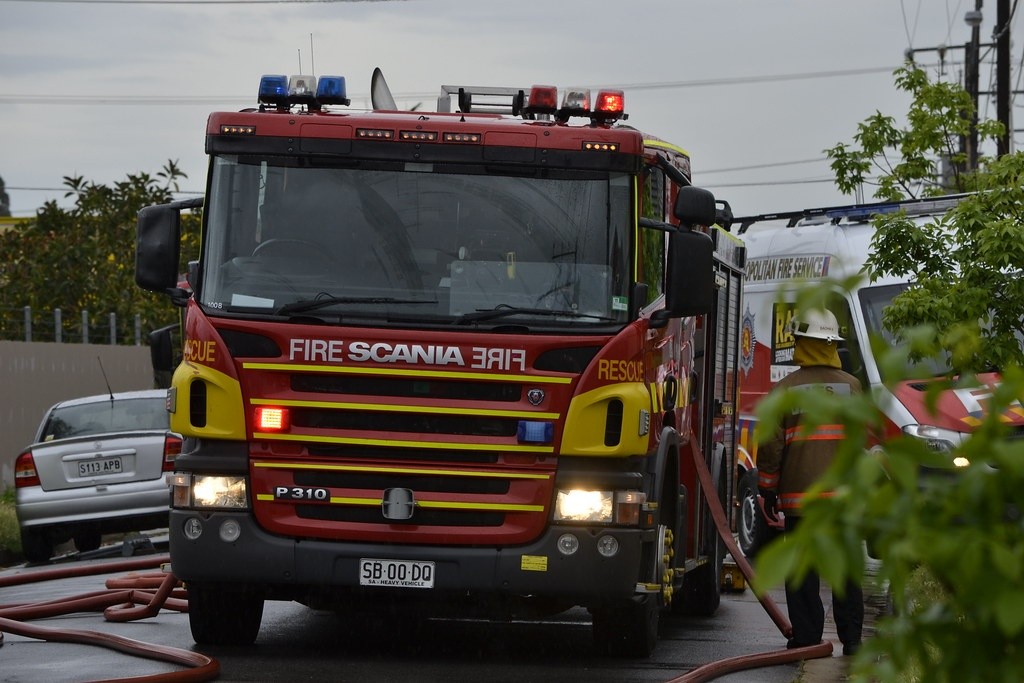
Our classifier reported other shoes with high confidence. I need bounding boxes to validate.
[843,642,862,655]
[787,638,798,649]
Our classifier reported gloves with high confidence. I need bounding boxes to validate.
[764,490,779,522]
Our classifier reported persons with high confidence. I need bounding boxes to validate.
[756,306,871,659]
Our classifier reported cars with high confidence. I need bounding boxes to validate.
[13,386,181,566]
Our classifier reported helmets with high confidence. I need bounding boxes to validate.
[789,307,846,345]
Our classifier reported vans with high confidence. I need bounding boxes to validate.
[733,190,1024,557]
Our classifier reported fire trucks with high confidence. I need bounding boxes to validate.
[135,65,747,651]
